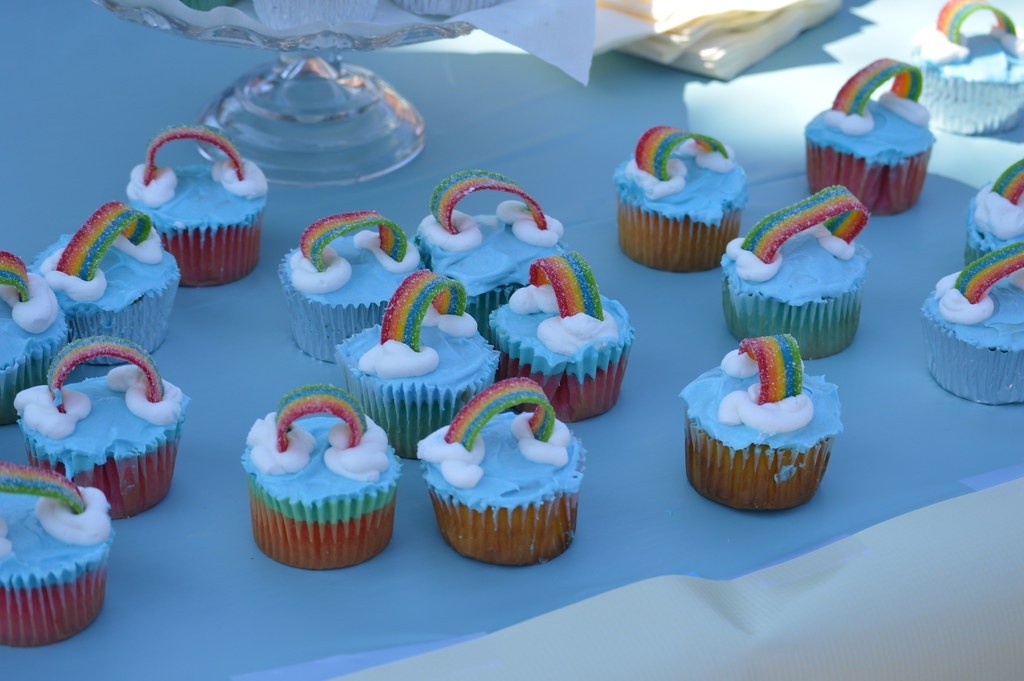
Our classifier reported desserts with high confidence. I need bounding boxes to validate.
[0,0,1024,648]
